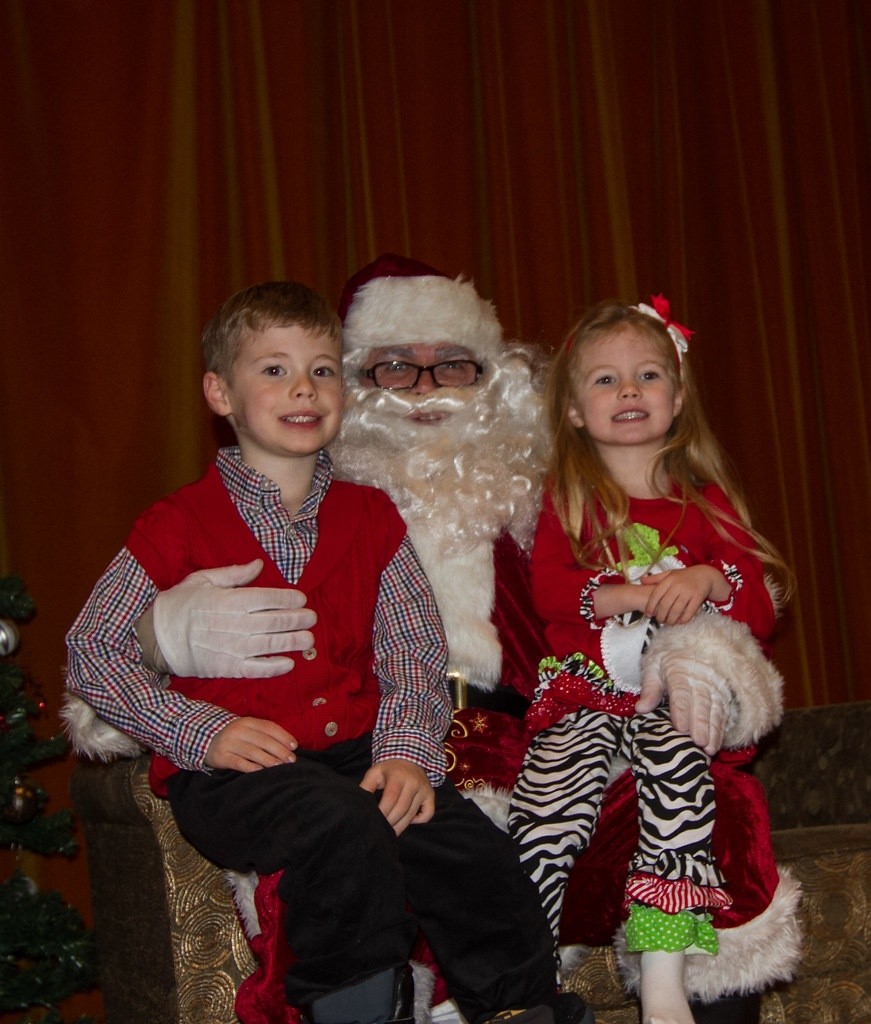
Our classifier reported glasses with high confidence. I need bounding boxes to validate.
[360,360,484,390]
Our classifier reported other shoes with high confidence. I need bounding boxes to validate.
[550,992,594,1024]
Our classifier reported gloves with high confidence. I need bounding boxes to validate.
[636,652,735,755]
[153,559,318,679]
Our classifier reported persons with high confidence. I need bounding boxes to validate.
[67,252,805,1024]
[509,296,796,1024]
[64,280,593,1024]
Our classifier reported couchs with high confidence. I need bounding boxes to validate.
[69,694,871,1024]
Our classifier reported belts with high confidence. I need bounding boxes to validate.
[447,676,533,719]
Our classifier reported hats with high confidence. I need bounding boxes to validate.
[337,252,504,377]
